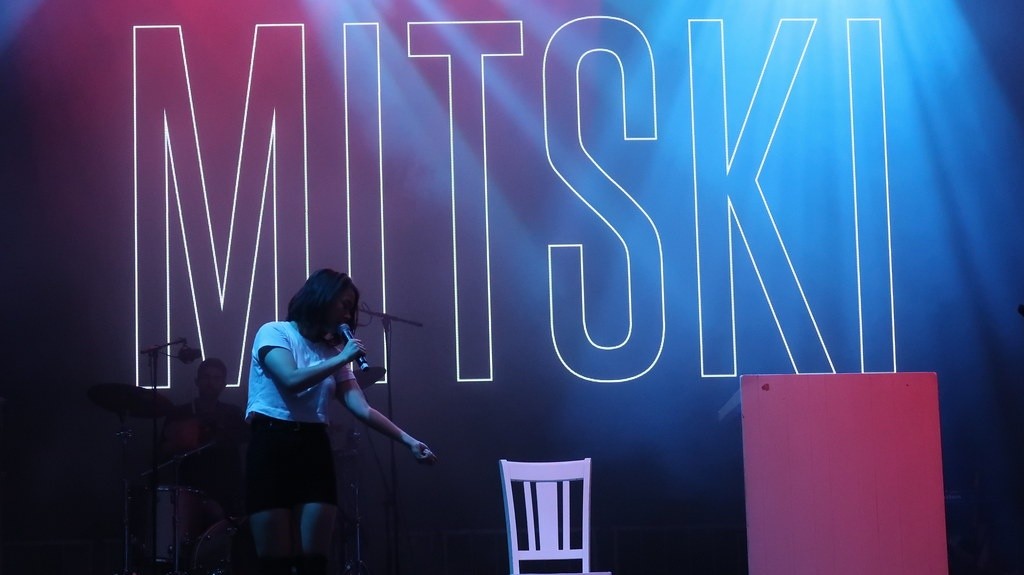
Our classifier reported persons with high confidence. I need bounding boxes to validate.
[160,358,242,499]
[231,269,438,575]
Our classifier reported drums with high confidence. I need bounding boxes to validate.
[125,485,207,567]
[187,514,260,575]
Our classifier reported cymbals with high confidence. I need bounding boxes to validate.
[77,382,178,429]
[354,365,387,390]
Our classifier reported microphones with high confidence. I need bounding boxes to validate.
[335,320,370,373]
[178,346,202,364]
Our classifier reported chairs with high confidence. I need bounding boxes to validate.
[500,458,612,575]
